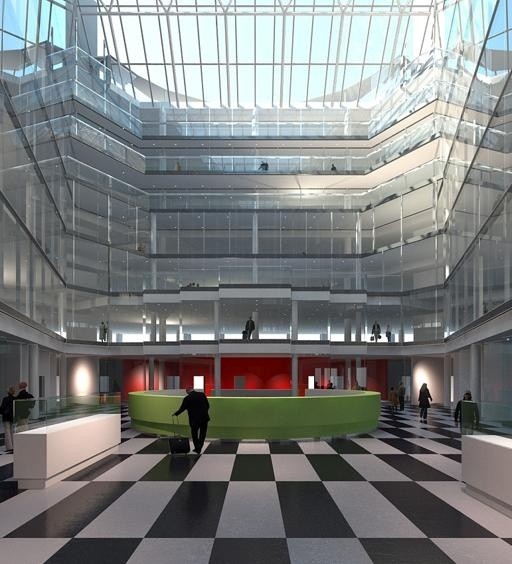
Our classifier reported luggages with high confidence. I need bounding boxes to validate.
[169,416,190,454]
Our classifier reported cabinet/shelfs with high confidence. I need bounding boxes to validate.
[67,326,97,341]
[14,413,121,488]
[461,434,512,508]
[414,326,443,344]
